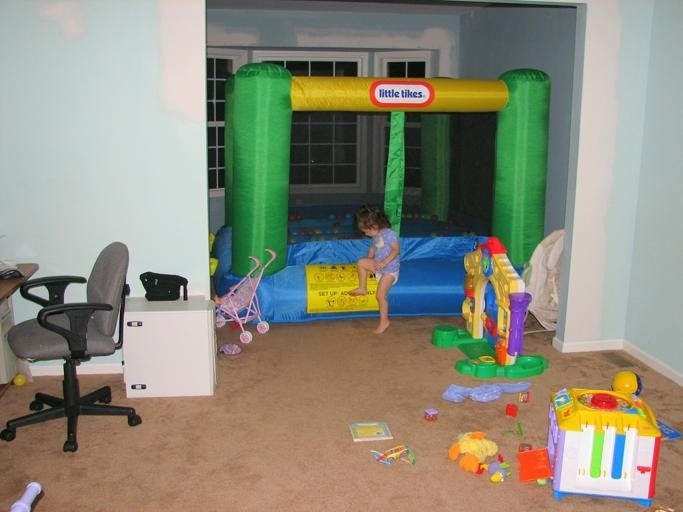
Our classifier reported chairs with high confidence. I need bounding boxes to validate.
[0,240,142,450]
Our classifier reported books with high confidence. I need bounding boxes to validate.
[349,422,393,441]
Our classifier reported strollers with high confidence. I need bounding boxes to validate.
[212,245,281,347]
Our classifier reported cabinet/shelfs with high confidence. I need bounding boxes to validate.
[120,296,216,399]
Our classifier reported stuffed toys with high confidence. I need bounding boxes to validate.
[448,429,497,472]
[480,455,511,483]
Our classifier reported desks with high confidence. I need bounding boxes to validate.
[0,260,39,316]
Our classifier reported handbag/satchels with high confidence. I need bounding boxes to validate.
[139,272,188,301]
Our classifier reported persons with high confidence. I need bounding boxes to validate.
[348,205,400,334]
